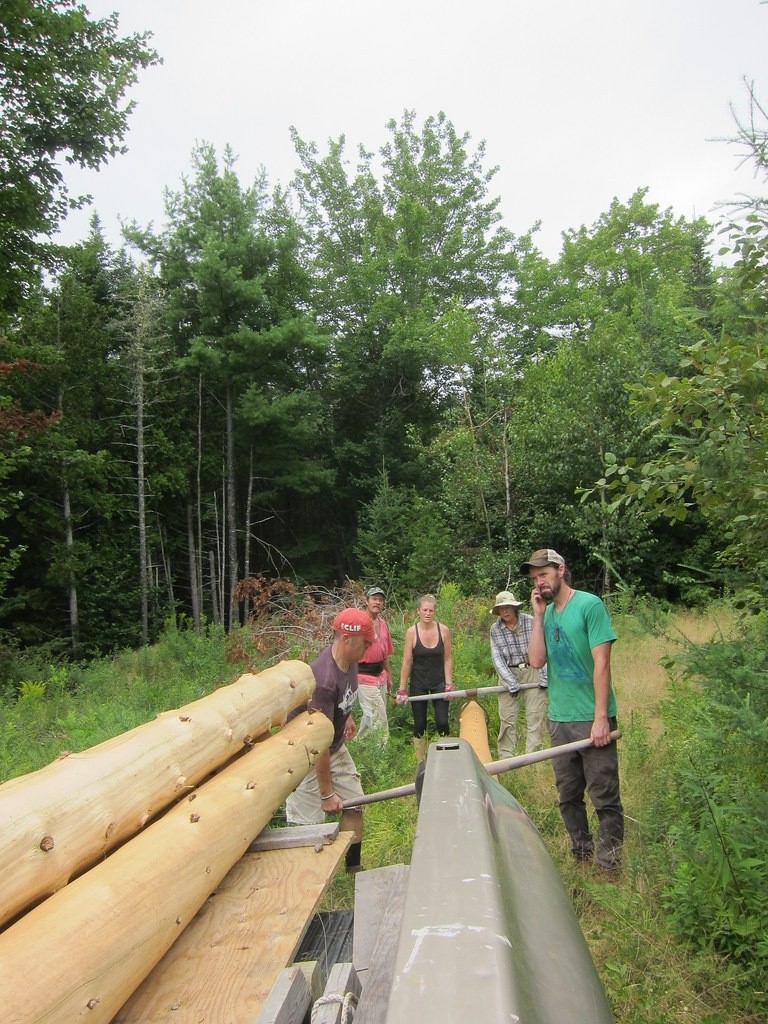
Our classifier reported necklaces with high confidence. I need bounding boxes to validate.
[551,590,571,641]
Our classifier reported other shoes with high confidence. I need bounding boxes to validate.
[591,859,622,883]
[568,848,594,864]
[345,865,364,875]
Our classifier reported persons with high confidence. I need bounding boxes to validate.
[395,594,455,762]
[285,608,375,876]
[354,587,394,750]
[520,548,625,882]
[488,590,548,761]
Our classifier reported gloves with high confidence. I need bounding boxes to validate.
[509,689,520,698]
[536,684,547,689]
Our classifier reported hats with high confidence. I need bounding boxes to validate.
[367,587,387,596]
[332,608,376,642]
[488,591,524,615]
[518,549,565,574]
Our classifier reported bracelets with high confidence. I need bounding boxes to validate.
[320,791,336,800]
